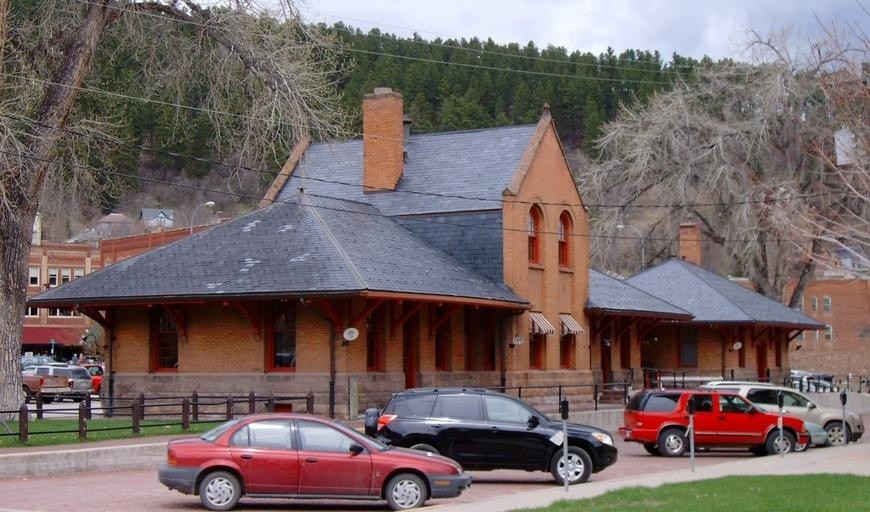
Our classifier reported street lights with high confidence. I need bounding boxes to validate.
[190,201,215,235]
[49,339,56,357]
[617,225,645,270]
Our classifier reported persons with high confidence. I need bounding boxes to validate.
[77,353,86,363]
[72,353,78,363]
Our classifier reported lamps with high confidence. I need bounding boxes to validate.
[79,334,108,350]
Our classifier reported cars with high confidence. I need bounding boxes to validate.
[21,356,106,403]
[63,231,103,243]
[791,370,839,392]
